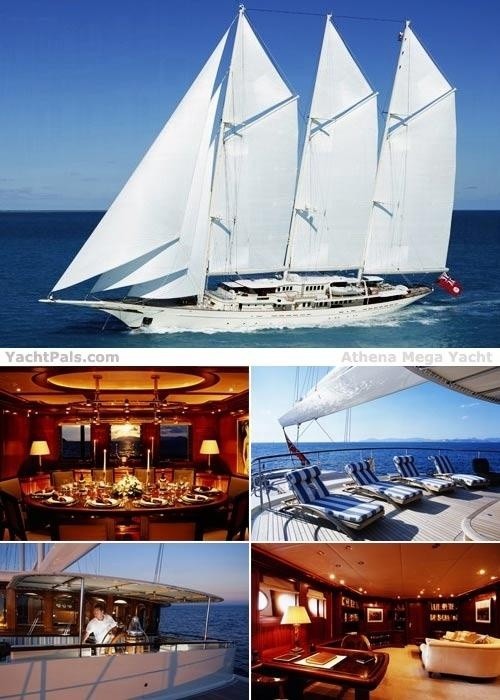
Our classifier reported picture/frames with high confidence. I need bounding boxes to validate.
[475,599,491,623]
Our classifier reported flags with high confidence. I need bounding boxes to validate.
[434,272,463,297]
[284,431,310,465]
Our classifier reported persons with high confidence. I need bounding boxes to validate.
[81,603,119,656]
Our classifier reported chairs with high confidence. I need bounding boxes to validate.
[341,633,371,651]
[428,455,491,491]
[0,468,249,541]
[278,465,385,539]
[389,456,456,496]
[343,461,423,511]
[472,458,500,487]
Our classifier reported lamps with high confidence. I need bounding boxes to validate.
[281,606,312,653]
[30,441,51,474]
[200,440,220,472]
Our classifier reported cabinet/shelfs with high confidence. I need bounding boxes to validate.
[334,590,462,648]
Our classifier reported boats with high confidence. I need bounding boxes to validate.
[255,366,499,541]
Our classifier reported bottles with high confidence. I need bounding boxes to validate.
[160,472,167,490]
[78,474,86,491]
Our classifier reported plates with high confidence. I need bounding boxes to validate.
[193,488,219,494]
[87,498,119,507]
[47,496,76,504]
[139,498,168,507]
[181,494,208,502]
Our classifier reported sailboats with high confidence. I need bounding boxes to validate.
[38,7,457,334]
[0,544,233,700]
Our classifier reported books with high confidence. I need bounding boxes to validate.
[272,653,302,662]
[356,655,374,664]
[342,596,360,622]
[306,652,337,665]
[429,602,458,623]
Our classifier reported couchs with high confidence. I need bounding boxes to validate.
[420,631,500,682]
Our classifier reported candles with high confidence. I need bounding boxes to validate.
[104,449,106,471]
[147,450,150,471]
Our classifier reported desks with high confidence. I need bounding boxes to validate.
[260,638,390,700]
